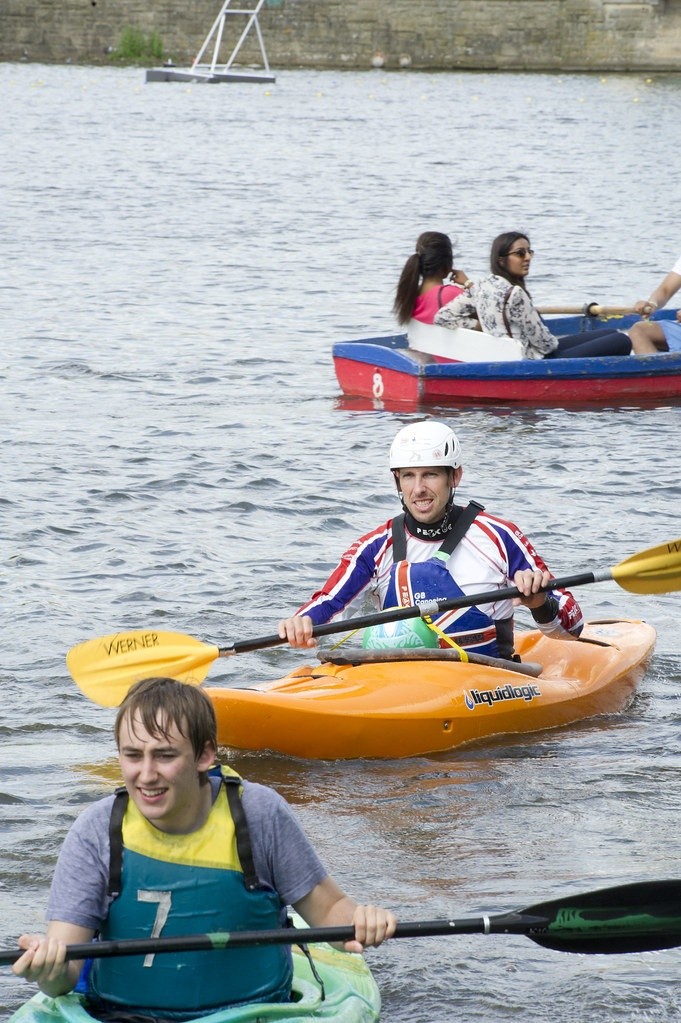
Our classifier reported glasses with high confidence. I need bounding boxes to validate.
[502,250,534,258]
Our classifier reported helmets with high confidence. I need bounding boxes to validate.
[389,421,462,471]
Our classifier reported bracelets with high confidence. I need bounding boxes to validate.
[650,301,658,305]
[464,280,472,287]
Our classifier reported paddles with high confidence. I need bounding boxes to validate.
[64,536,681,713]
[0,875,681,966]
[536,303,653,316]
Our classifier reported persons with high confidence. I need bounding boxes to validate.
[433,231,632,360]
[14,678,399,1023]
[629,257,681,354]
[391,231,475,363]
[278,421,584,660]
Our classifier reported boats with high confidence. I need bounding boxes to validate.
[200,618,657,760]
[333,308,681,405]
[3,913,382,1023]
